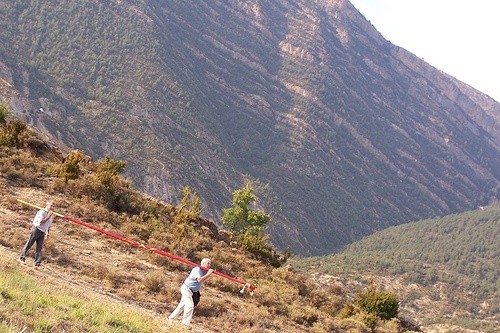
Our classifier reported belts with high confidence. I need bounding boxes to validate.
[183,282,193,293]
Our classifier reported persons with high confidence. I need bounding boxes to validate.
[168,258,214,329]
[19,200,57,270]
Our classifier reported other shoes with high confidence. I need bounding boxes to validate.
[20,257,26,262]
[166,316,173,322]
[182,322,192,330]
[35,261,43,266]
